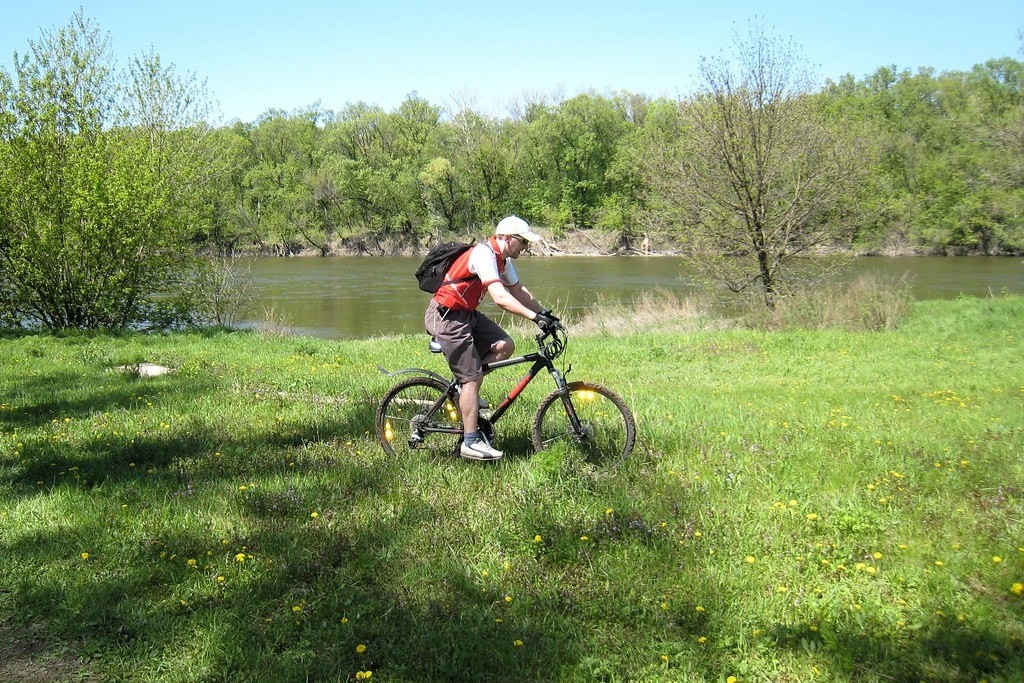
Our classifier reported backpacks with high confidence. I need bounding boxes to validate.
[415,237,495,293]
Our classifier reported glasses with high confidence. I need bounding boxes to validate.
[511,235,529,244]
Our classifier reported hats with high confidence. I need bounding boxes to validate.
[497,216,540,241]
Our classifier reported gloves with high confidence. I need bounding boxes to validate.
[538,309,559,323]
[532,313,552,327]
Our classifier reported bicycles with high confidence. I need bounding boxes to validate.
[373,318,636,475]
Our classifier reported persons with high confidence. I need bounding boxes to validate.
[414,215,560,461]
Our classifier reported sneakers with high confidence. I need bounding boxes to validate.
[460,438,503,460]
[457,386,489,408]
